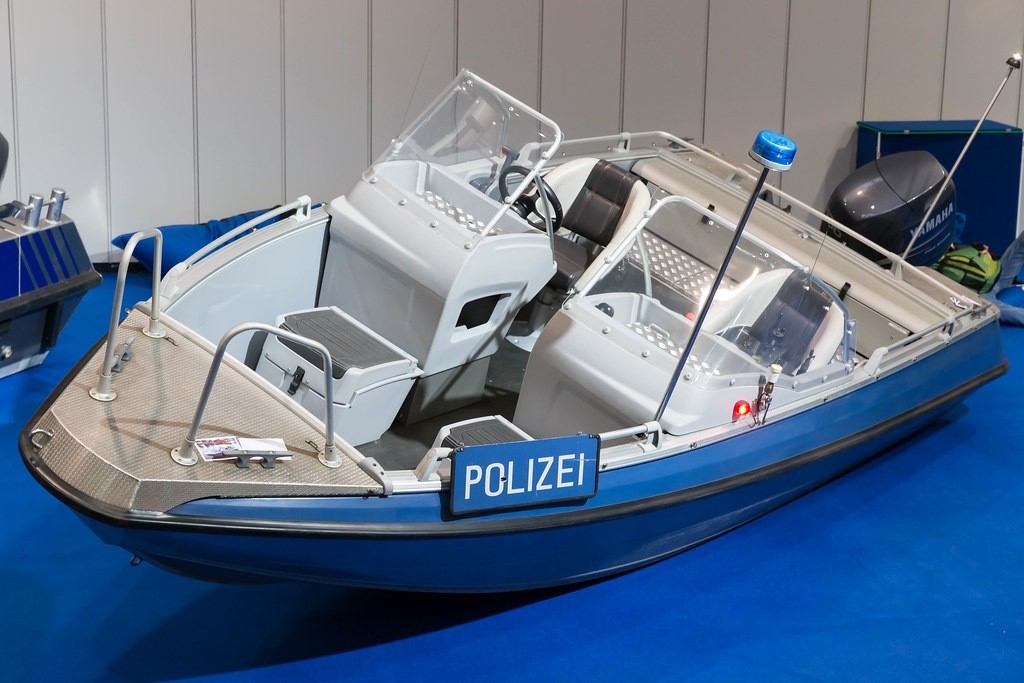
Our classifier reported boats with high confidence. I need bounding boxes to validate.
[16,50,1020,602]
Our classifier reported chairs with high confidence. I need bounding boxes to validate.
[700,269,846,373]
[497,156,651,347]
